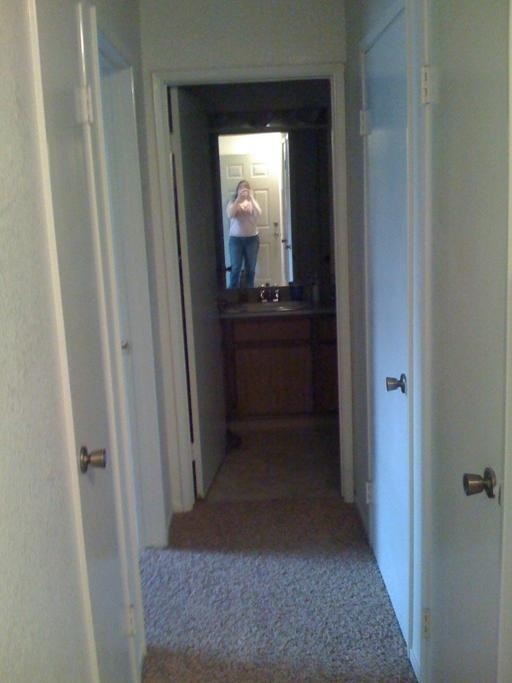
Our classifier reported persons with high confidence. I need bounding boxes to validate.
[226,180,262,288]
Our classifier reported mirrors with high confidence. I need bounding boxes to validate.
[215,131,293,290]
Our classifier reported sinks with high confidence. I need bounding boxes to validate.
[245,302,304,310]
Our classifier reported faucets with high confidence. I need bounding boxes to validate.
[273,290,280,302]
[260,289,268,303]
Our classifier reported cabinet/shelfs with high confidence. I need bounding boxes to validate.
[313,313,337,415]
[229,315,313,417]
